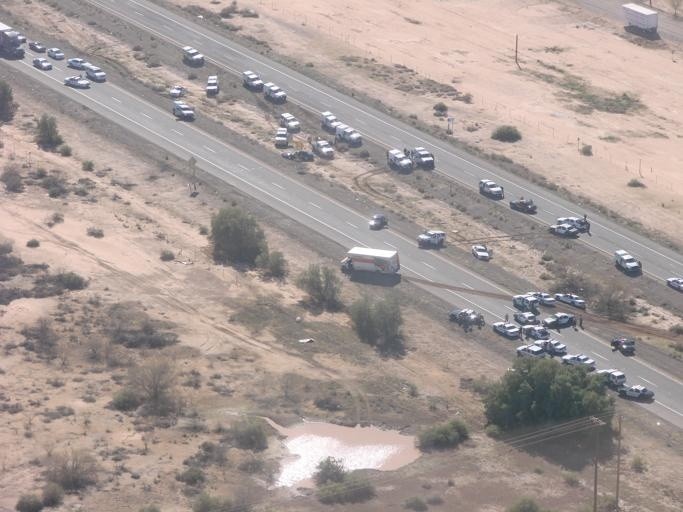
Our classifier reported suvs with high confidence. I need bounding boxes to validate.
[418,229,445,246]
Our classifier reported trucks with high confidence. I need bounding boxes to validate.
[480,179,503,199]
[241,70,263,90]
[623,3,657,33]
[206,76,220,96]
[387,146,435,171]
[589,369,625,388]
[264,82,286,103]
[615,250,639,274]
[274,109,362,158]
[182,46,203,64]
[170,84,195,119]
[341,246,400,276]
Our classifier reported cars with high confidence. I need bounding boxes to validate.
[451,310,484,325]
[510,199,536,213]
[369,212,387,230]
[493,291,594,369]
[611,339,635,353]
[617,384,654,400]
[0,22,105,89]
[668,277,683,291]
[551,216,588,235]
[472,245,492,261]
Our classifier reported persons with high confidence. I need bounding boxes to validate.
[504,312,509,322]
[579,316,584,329]
[571,317,577,328]
[519,325,523,338]
